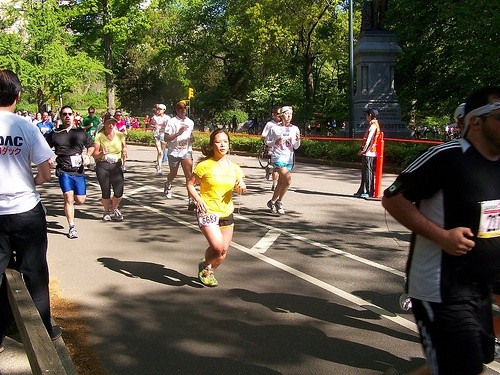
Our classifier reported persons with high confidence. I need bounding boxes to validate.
[185,130,248,288]
[163,102,197,210]
[0,69,62,353]
[92,115,128,223]
[353,108,380,198]
[15,104,462,175]
[42,105,95,239]
[381,87,500,375]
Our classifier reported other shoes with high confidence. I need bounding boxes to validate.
[155,173,162,178]
[188,201,197,211]
[354,193,361,197]
[163,181,172,199]
[399,292,413,312]
[271,186,276,191]
[52,325,63,340]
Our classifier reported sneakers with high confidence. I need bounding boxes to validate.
[267,200,276,213]
[68,225,78,239]
[113,209,124,220]
[274,199,285,214]
[198,262,211,287]
[210,271,218,287]
[102,213,111,221]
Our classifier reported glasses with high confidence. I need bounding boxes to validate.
[480,115,500,120]
[89,112,94,113]
[62,113,72,116]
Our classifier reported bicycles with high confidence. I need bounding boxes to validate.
[257,144,296,171]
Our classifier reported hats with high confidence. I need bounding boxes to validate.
[152,104,159,110]
[453,103,466,124]
[363,109,378,116]
[102,112,118,122]
[158,104,166,110]
